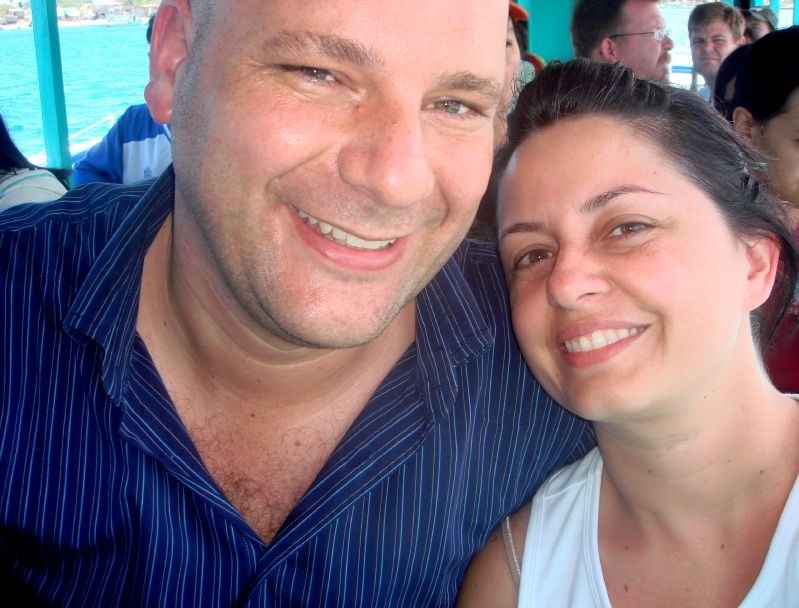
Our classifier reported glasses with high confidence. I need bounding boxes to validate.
[600,29,671,55]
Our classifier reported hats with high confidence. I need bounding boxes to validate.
[741,7,778,32]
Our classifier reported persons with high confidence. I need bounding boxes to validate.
[0,0,598,608]
[455,56,799,608]
[502,0,799,394]
[0,16,173,220]
